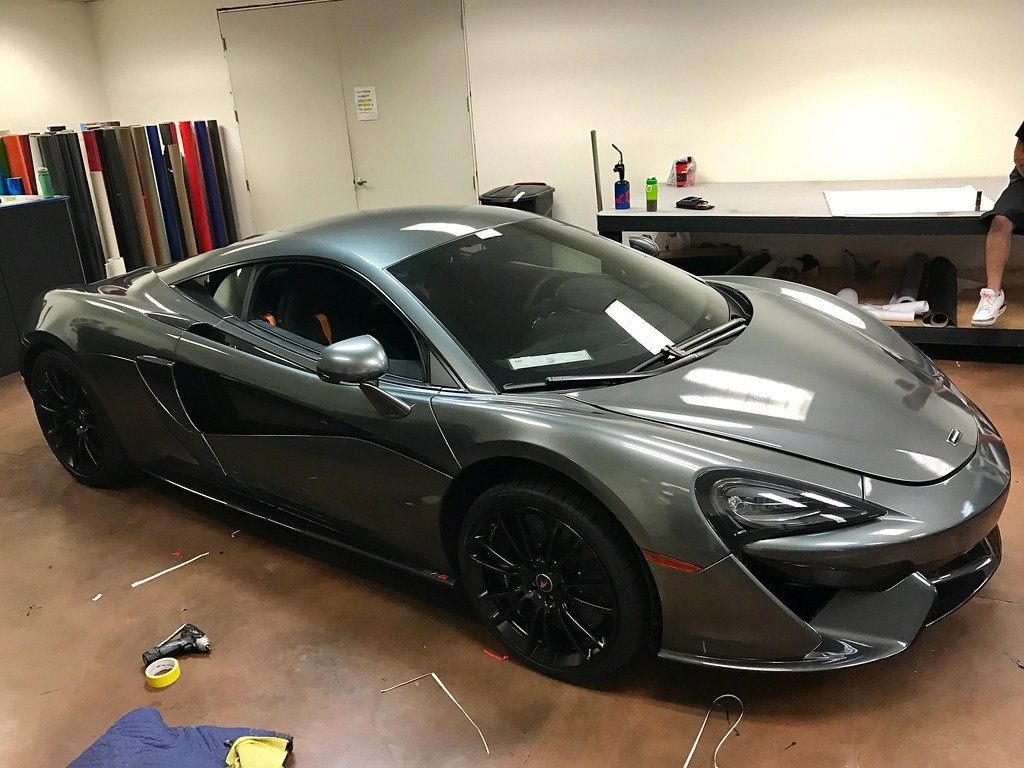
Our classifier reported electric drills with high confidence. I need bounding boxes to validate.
[142,623,212,665]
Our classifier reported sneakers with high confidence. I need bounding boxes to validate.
[972,288,1008,326]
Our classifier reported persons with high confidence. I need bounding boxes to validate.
[971,120,1024,327]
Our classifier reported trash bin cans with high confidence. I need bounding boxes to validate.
[480,183,555,217]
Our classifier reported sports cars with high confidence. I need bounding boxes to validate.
[18,202,1012,693]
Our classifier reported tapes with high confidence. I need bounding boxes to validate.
[145,657,181,687]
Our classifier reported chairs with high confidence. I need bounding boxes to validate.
[283,266,400,359]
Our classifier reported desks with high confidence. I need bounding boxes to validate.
[596,177,1024,347]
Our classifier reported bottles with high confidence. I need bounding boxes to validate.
[37,166,54,199]
[675,156,692,187]
[646,177,658,212]
